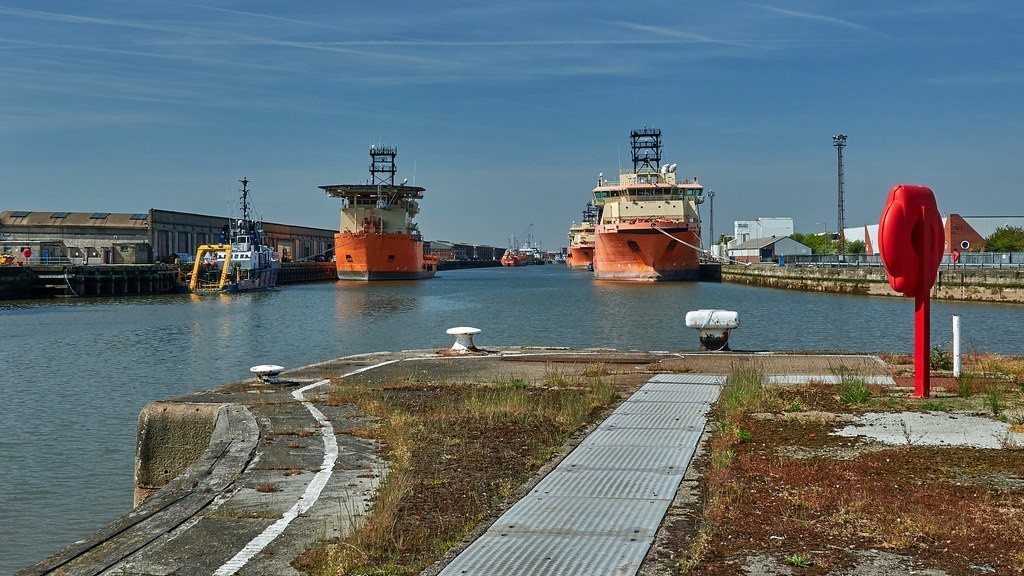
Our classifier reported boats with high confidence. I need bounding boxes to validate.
[177,177,281,295]
[318,139,442,281]
[499,234,567,268]
[590,124,705,283]
[563,199,603,271]
